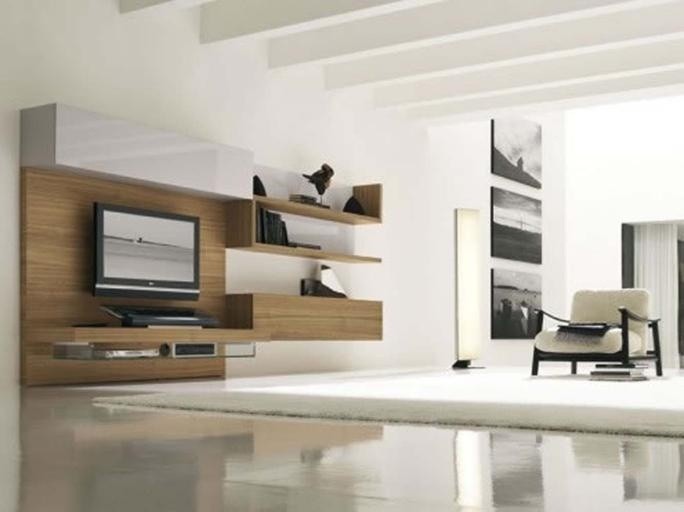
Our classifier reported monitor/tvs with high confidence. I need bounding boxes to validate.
[91,201,201,301]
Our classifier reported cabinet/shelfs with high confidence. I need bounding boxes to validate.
[21,168,382,386]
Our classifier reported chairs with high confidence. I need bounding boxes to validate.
[531,308,662,375]
